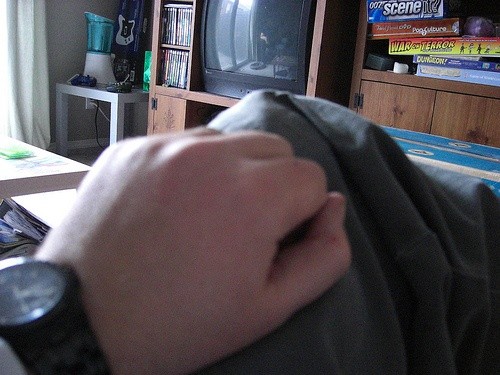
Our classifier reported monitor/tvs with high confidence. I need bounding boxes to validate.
[199,0,317,100]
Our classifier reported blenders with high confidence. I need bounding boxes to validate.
[84,11,117,83]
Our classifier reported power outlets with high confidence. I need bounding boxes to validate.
[85,97,97,110]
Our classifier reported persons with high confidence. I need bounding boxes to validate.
[1,89,500,375]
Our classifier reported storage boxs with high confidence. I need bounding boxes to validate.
[381,125,500,198]
[412,54,500,87]
[366,17,464,40]
[388,36,500,58]
[366,0,448,24]
[365,53,394,70]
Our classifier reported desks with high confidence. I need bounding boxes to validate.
[1,134,91,199]
[56,82,149,158]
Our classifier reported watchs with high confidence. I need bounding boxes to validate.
[0,257,110,374]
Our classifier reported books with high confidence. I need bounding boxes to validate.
[159,4,193,90]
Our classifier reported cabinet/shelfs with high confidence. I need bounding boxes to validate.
[147,0,500,149]
